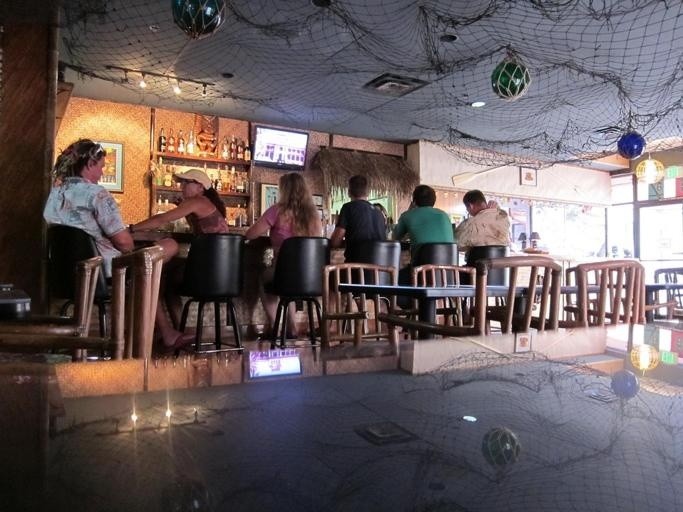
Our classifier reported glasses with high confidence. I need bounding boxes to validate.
[96,145,104,155]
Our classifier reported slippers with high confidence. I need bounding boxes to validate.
[159,332,195,354]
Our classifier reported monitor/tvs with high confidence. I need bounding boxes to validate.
[244,345,303,380]
[252,124,310,171]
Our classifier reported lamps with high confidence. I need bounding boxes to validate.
[628,341,661,371]
[617,105,646,160]
[171,0,227,39]
[491,44,532,100]
[635,138,667,185]
[613,371,640,413]
[159,472,210,512]
[480,426,520,481]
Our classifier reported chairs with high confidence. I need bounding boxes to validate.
[2,235,167,361]
[319,252,682,356]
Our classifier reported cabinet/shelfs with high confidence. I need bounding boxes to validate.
[151,107,253,226]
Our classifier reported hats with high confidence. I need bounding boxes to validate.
[173,169,211,190]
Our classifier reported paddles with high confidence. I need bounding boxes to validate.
[451,161,510,187]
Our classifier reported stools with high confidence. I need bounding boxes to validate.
[173,233,509,352]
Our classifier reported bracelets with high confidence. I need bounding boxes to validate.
[129,224,135,234]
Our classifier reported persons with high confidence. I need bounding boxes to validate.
[374,203,391,240]
[453,190,510,324]
[392,185,455,331]
[329,176,387,334]
[244,173,321,341]
[44,139,197,354]
[122,170,230,331]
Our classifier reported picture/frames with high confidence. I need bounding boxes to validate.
[519,167,538,186]
[86,141,124,194]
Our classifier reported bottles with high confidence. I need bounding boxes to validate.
[154,195,172,214]
[157,127,251,161]
[227,196,248,228]
[155,158,246,193]
